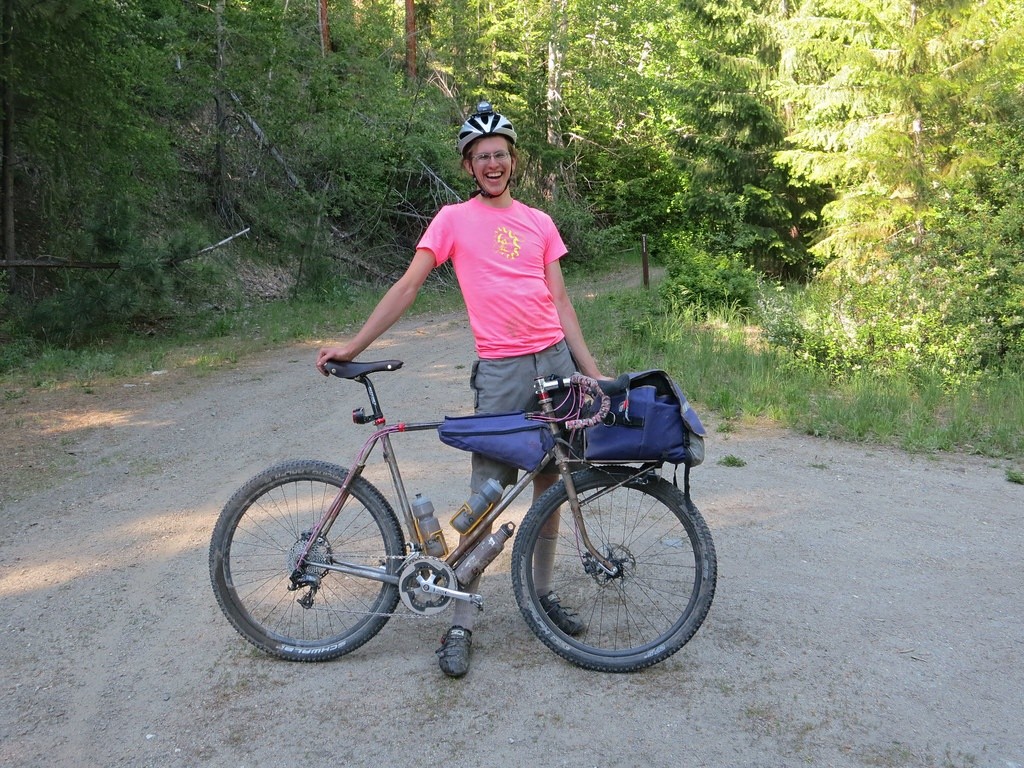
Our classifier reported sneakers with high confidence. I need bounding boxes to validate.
[435,624,472,677]
[538,590,585,635]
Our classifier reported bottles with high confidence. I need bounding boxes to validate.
[411,492,448,558]
[449,478,503,534]
[454,520,517,587]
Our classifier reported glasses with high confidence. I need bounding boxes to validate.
[470,150,511,162]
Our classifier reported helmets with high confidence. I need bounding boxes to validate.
[457,102,517,155]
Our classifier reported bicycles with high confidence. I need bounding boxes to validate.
[209,358,717,674]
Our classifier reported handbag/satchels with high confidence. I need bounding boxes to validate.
[584,366,707,466]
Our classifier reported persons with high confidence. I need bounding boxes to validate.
[318,108,622,679]
